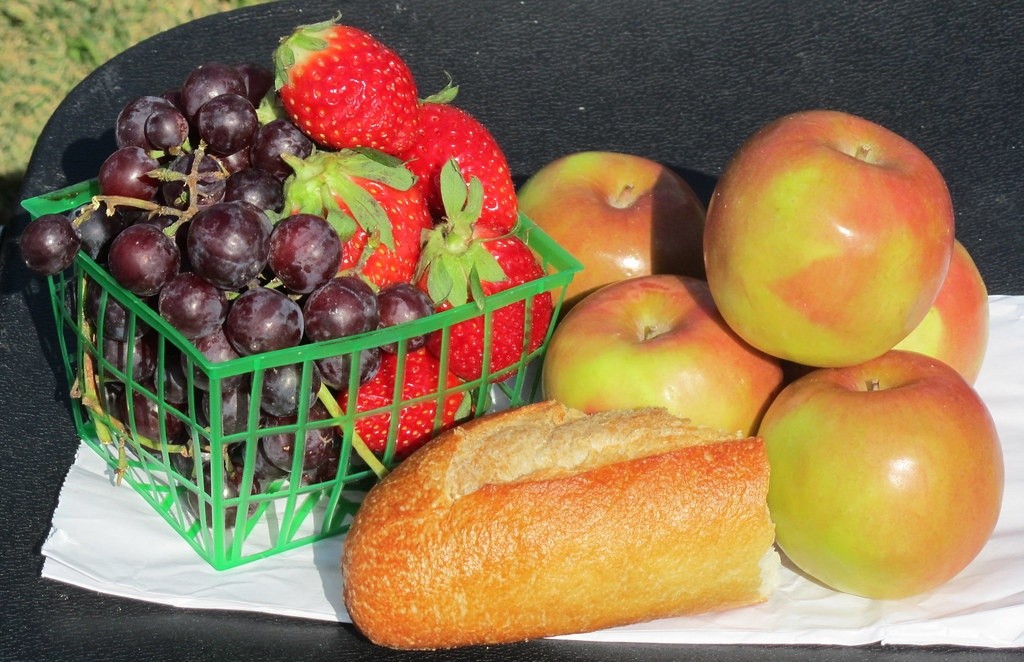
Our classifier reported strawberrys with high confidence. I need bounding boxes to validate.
[274,9,555,459]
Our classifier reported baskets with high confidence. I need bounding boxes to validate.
[19,177,583,571]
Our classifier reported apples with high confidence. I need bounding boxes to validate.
[539,272,786,439]
[889,236,990,389]
[753,348,1005,601]
[701,109,954,371]
[513,148,707,317]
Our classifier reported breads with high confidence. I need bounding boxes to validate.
[340,397,779,651]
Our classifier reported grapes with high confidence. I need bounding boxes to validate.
[21,63,438,529]
